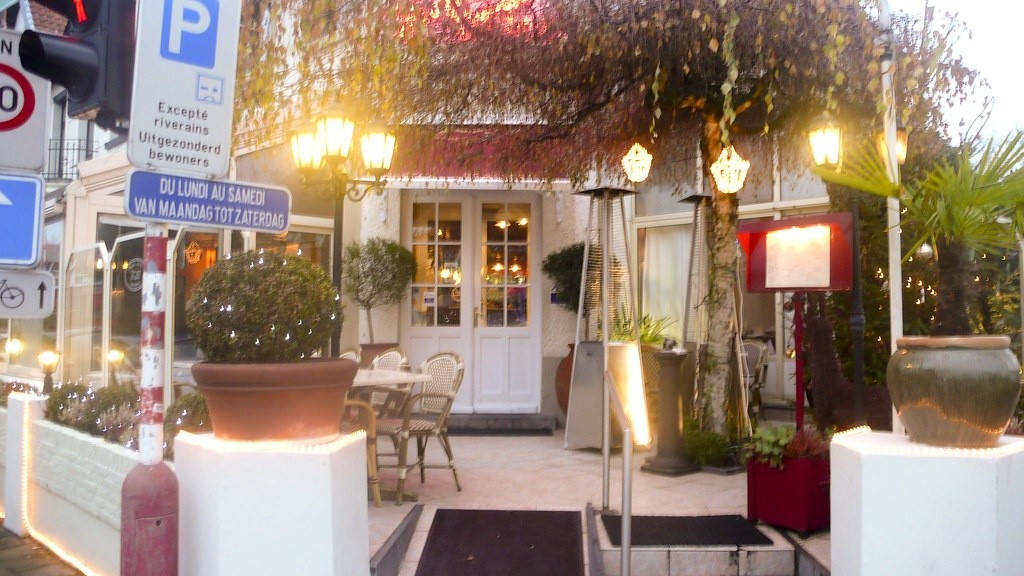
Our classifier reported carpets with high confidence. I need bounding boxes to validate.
[601,515,774,545]
[414,509,585,576]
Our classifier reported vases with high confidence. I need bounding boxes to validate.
[192,356,358,446]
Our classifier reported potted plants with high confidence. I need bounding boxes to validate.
[536,242,622,420]
[729,423,840,541]
[803,123,1024,448]
[339,237,417,368]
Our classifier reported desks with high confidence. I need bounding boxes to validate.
[351,369,433,483]
[308,346,464,508]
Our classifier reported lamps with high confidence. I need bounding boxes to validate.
[37,343,60,395]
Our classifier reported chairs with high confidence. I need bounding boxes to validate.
[735,339,767,427]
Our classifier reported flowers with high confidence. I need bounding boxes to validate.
[185,248,343,362]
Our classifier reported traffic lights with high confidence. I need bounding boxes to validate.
[18,0,136,121]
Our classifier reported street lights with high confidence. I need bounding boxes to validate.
[290,108,397,359]
[806,113,909,427]
